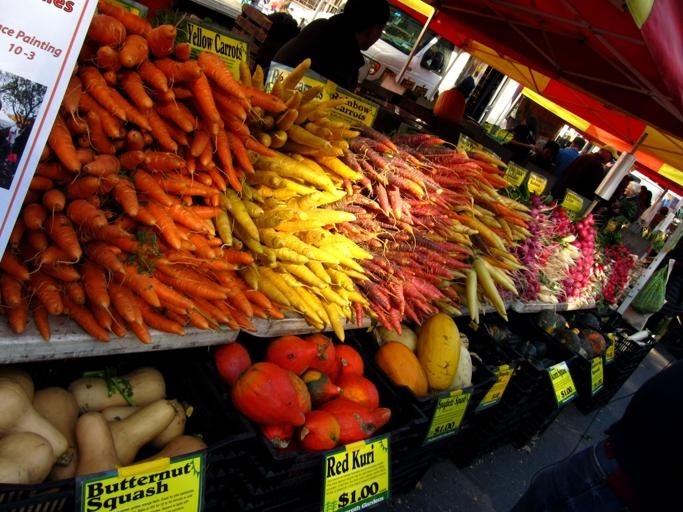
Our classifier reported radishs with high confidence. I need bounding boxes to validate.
[535,234,581,304]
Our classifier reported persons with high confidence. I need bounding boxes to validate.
[500,112,669,226]
[255,0,390,98]
[506,353,683,512]
[432,78,475,124]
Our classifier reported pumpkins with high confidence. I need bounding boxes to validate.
[216,335,391,451]
[537,305,606,360]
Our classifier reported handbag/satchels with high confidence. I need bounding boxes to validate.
[630,264,668,312]
[0,310,657,512]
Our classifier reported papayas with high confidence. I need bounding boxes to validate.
[374,312,473,398]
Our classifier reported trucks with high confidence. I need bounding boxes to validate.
[354,3,454,102]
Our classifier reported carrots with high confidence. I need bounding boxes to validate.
[0,1,534,344]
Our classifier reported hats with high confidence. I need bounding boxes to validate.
[602,144,619,160]
